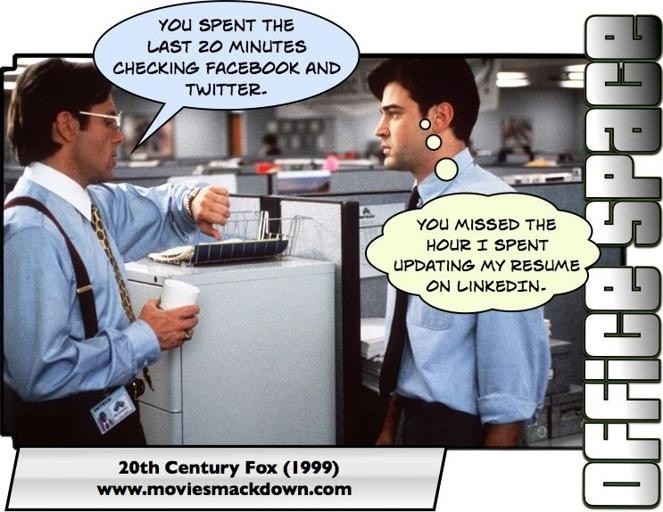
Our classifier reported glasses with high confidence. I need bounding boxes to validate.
[81,108,125,132]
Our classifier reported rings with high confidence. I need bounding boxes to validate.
[184,331,191,341]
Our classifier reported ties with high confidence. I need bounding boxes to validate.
[379,188,420,402]
[89,206,156,392]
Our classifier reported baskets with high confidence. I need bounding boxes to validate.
[152,208,302,267]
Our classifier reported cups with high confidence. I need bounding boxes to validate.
[158,279,201,310]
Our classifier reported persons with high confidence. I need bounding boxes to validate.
[257,132,284,156]
[1,57,231,447]
[362,54,552,447]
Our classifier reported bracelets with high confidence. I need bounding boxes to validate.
[183,186,200,219]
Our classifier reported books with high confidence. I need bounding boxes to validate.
[159,237,251,257]
[148,248,194,263]
